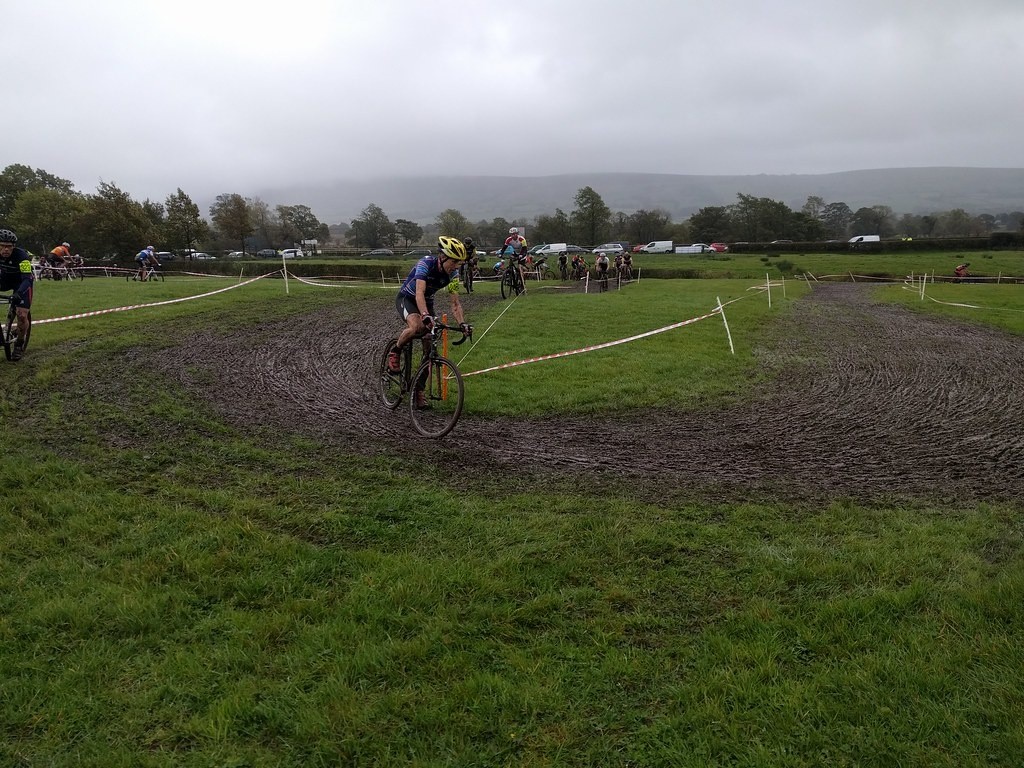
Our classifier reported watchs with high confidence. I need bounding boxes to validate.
[420,311,429,319]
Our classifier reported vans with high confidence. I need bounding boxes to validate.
[847,235,880,241]
[644,241,673,254]
[536,244,623,255]
[155,252,173,259]
[772,240,793,244]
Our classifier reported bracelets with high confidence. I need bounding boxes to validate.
[459,322,465,326]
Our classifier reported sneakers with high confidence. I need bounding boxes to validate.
[387,349,403,374]
[11,337,25,361]
[415,390,430,410]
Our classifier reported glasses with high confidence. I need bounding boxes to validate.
[447,259,465,266]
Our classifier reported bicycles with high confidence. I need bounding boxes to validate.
[379,315,472,439]
[0,293,32,362]
[473,266,556,282]
[560,265,632,293]
[952,272,976,284]
[39,261,83,281]
[459,254,525,299]
[127,259,164,284]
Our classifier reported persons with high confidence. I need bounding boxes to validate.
[0,230,34,357]
[135,246,161,281]
[521,251,633,291]
[39,242,84,281]
[498,227,527,294]
[486,245,503,257]
[250,249,317,258]
[701,244,705,252]
[954,263,972,278]
[492,260,505,276]
[459,237,478,292]
[387,236,471,407]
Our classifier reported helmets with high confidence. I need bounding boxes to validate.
[508,228,519,234]
[560,252,565,256]
[464,236,472,244]
[600,253,607,257]
[62,241,71,249]
[435,235,467,261]
[146,245,155,251]
[500,259,505,263]
[0,229,18,246]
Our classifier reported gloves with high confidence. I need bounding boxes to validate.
[10,292,26,307]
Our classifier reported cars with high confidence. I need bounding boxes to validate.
[527,243,591,257]
[475,246,514,256]
[185,252,256,259]
[633,244,645,252]
[361,248,432,256]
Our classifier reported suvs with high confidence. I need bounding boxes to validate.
[256,248,304,258]
[691,243,729,253]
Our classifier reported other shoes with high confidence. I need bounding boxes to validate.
[470,285,474,291]
